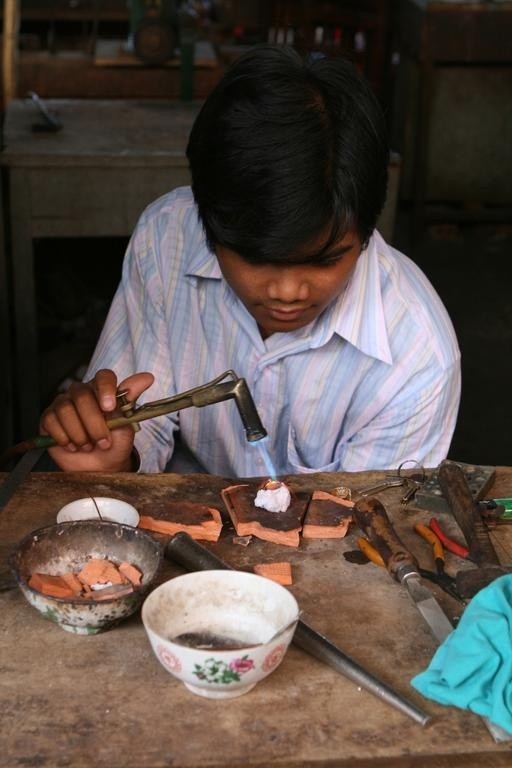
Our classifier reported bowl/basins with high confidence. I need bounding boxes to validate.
[141,570,300,698]
[56,496,140,531]
[8,520,164,637]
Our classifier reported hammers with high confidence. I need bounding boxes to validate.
[439,465,511,598]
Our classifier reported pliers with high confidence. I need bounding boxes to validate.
[358,524,467,608]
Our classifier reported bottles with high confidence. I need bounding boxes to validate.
[266,19,368,57]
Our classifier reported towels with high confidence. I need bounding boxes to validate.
[410,572,512,735]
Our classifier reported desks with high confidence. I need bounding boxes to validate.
[5,98,400,439]
[0,469,512,766]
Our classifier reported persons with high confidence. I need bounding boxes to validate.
[39,44,462,474]
